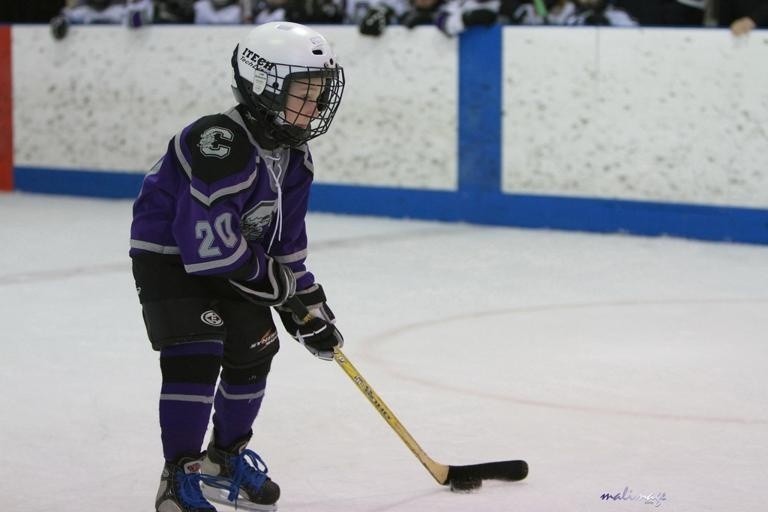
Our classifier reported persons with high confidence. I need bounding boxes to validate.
[50,0,768,35]
[129,22,344,512]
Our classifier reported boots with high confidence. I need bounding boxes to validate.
[154,452,219,512]
[201,412,280,505]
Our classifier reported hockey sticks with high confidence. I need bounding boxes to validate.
[286,294,529,486]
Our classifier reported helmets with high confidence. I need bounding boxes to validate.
[229,21,346,151]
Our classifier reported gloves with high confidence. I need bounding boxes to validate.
[51,14,71,41]
[360,4,396,36]
[228,251,296,309]
[462,8,498,28]
[128,9,150,31]
[273,282,344,361]
